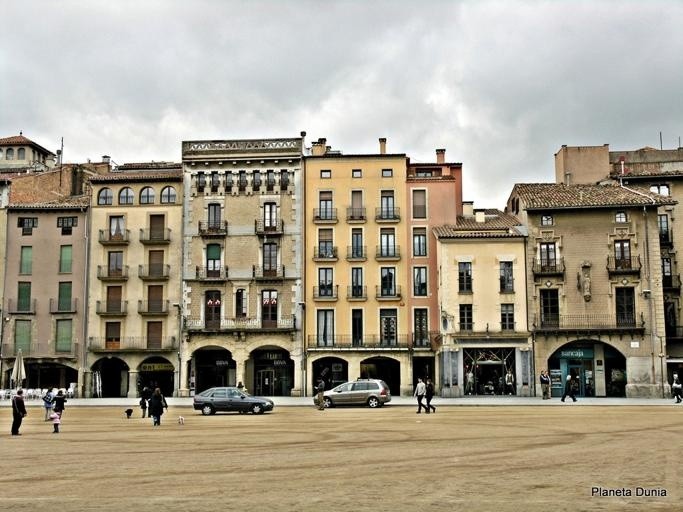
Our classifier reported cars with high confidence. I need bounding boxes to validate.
[314,377,391,409]
[194,386,273,415]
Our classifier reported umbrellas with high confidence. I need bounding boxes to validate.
[10,350,27,388]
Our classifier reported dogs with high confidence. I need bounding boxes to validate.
[177,416,184,424]
[125,409,133,419]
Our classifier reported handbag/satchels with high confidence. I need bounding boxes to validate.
[19,409,27,417]
[671,382,682,389]
[161,399,166,408]
[42,394,52,403]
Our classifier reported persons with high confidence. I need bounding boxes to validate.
[236,382,247,415]
[544,371,551,399]
[540,371,549,399]
[560,375,578,402]
[425,378,437,414]
[138,387,151,418]
[413,377,429,414]
[505,369,514,396]
[313,377,326,411]
[51,390,67,421]
[48,408,61,433]
[672,371,683,404]
[465,369,474,395]
[148,388,169,427]
[10,389,27,435]
[43,387,55,421]
